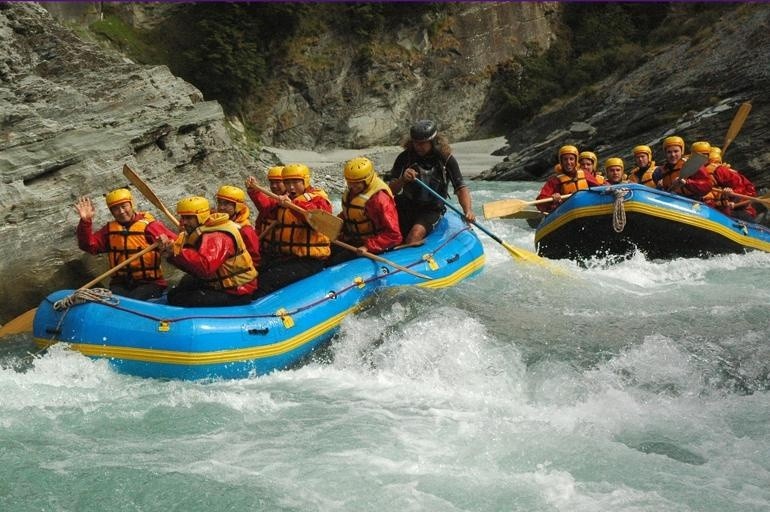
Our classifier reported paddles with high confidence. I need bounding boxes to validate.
[482,194,572,220]
[501,211,549,220]
[333,241,432,281]
[123,164,186,232]
[667,151,708,192]
[720,103,751,158]
[712,188,770,209]
[250,179,343,240]
[414,173,542,265]
[0,241,161,338]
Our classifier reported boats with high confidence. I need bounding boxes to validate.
[31,213,485,383]
[533,183,766,261]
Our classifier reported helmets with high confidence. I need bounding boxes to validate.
[106,188,134,219]
[708,152,721,163]
[177,196,211,226]
[558,145,578,167]
[605,157,624,177]
[215,186,245,214]
[281,163,310,188]
[631,145,652,163]
[344,157,374,187]
[579,151,597,172]
[410,120,437,143]
[268,166,284,181]
[711,146,722,154]
[663,136,684,157]
[691,141,710,160]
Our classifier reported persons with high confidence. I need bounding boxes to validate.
[74,189,166,301]
[245,164,334,280]
[338,156,403,257]
[628,146,662,189]
[536,145,601,213]
[209,186,261,271]
[155,196,259,307]
[659,136,714,202]
[597,158,629,184]
[555,151,610,186]
[711,147,757,218]
[684,142,744,200]
[385,120,475,243]
[256,167,285,231]
